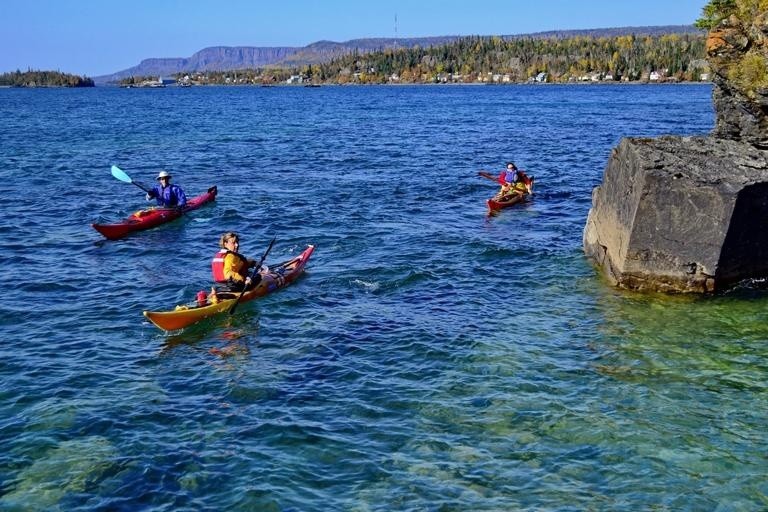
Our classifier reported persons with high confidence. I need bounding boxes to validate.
[495,162,518,197]
[144,170,186,213]
[209,230,271,292]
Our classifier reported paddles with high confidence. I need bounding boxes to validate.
[479,172,523,197]
[111,165,212,222]
[228,232,276,315]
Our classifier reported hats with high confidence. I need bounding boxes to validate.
[156,171,172,180]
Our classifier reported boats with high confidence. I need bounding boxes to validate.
[143,244,315,337]
[484,175,534,212]
[88,184,218,239]
